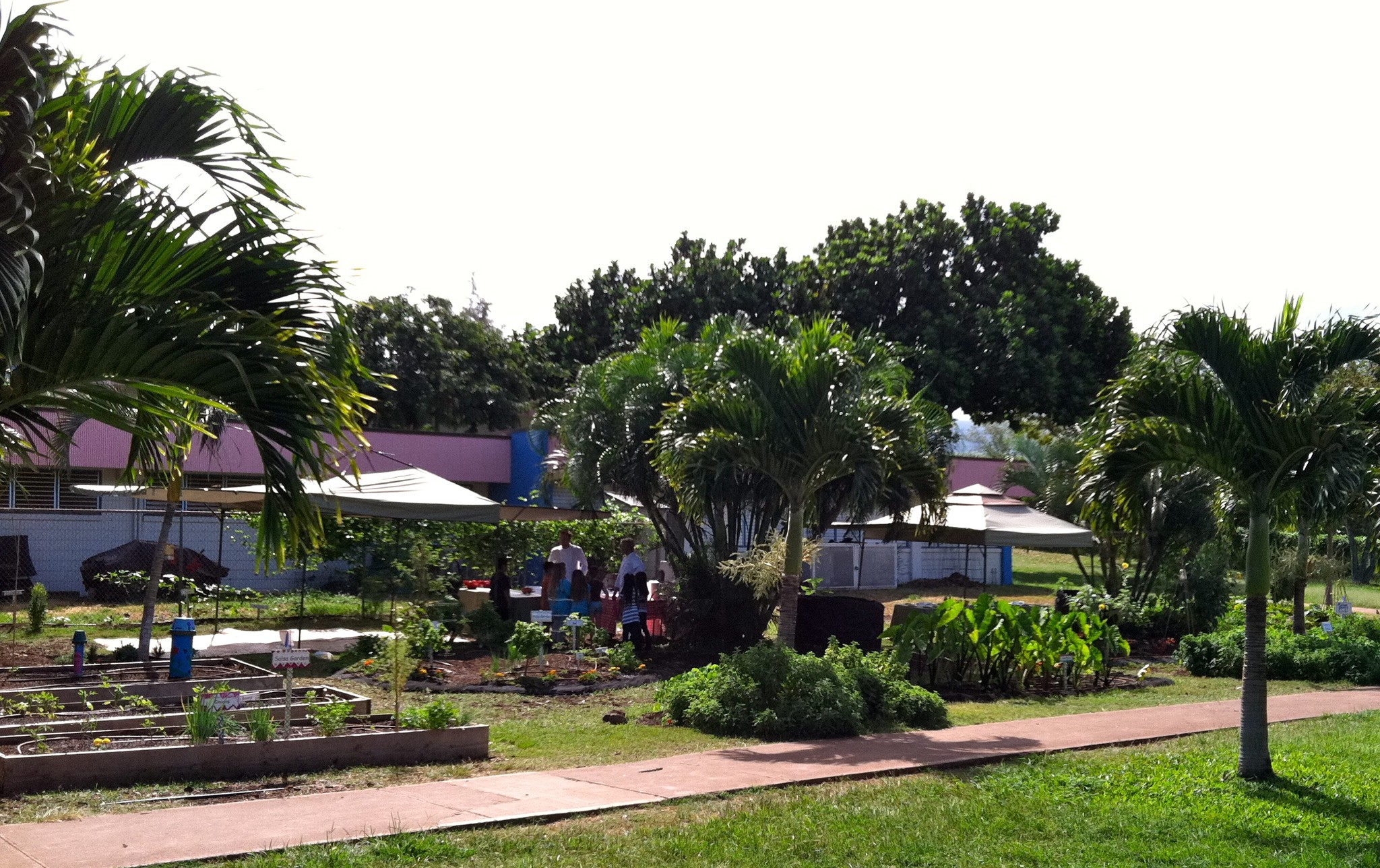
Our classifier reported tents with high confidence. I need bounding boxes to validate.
[857,483,1095,600]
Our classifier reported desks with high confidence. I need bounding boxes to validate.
[459,589,543,630]
[593,594,665,639]
[890,601,1039,629]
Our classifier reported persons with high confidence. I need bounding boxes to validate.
[612,539,651,653]
[489,559,511,622]
[540,561,609,642]
[548,529,589,581]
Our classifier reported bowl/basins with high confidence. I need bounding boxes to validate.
[464,580,490,589]
[522,588,532,594]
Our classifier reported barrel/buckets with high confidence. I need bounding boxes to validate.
[549,598,574,653]
